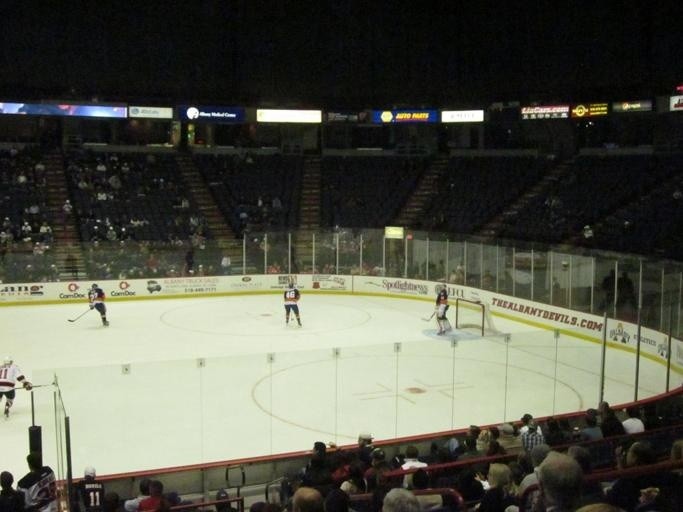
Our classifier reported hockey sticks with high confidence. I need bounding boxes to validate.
[421,311,435,322]
[69,309,92,322]
[15,382,55,389]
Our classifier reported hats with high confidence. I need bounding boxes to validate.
[359,432,374,440]
[366,447,384,459]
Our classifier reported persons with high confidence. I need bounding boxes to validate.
[433,283,451,335]
[17,452,62,512]
[0,470,24,512]
[72,467,239,512]
[86,283,110,328]
[0,358,32,420]
[282,282,303,329]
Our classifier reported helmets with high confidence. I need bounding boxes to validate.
[83,465,96,477]
[2,356,13,364]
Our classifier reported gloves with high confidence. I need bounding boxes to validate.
[22,382,32,391]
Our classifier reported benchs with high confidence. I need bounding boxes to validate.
[0,147,225,285]
[447,149,683,340]
[169,401,683,511]
[224,148,446,282]
